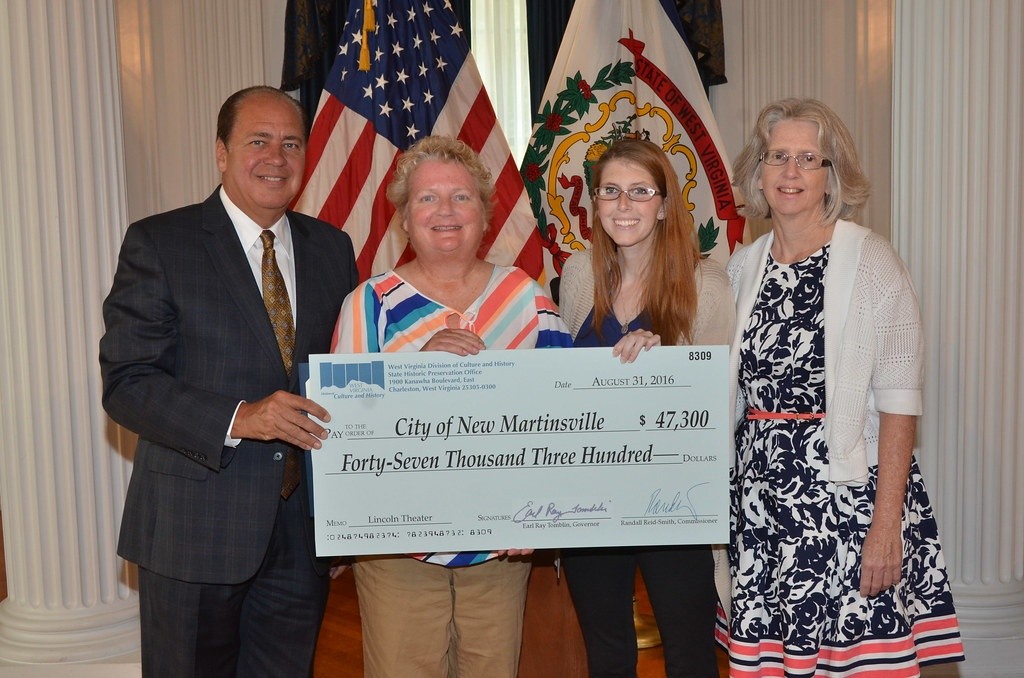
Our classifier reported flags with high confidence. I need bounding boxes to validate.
[283,0,759,304]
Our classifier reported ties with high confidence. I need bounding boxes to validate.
[259,230,298,501]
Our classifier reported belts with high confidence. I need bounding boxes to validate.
[747,406,827,420]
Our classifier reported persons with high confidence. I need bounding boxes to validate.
[96,84,361,678]
[708,98,971,678]
[555,135,736,678]
[326,134,576,678]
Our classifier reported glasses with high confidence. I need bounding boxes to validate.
[593,185,663,201]
[759,150,831,170]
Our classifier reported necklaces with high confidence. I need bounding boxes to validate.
[619,281,644,335]
